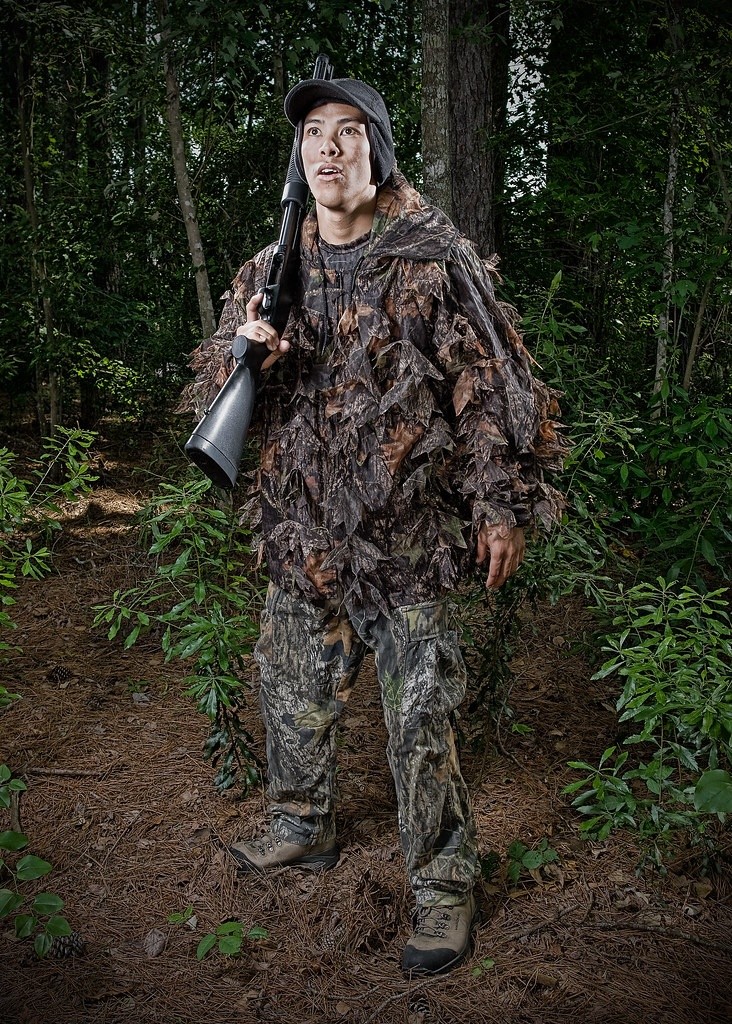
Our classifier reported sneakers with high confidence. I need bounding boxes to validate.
[402,895,480,975]
[225,831,341,874]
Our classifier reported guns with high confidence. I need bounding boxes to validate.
[183,52,338,491]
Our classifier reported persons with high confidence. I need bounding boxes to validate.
[174,77,566,978]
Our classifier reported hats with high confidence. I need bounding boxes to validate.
[285,75,394,187]
